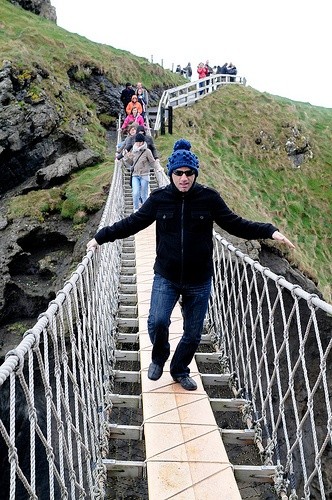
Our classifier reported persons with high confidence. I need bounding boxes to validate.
[87,139,295,391]
[115,82,164,214]
[176,60,237,95]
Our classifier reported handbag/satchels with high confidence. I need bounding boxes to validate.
[129,165,134,187]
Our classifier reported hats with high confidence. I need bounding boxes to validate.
[135,133,145,142]
[166,138,200,177]
[136,126,145,134]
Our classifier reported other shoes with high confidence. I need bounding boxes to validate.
[173,374,198,391]
[147,361,165,381]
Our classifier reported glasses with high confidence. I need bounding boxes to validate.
[172,169,196,177]
